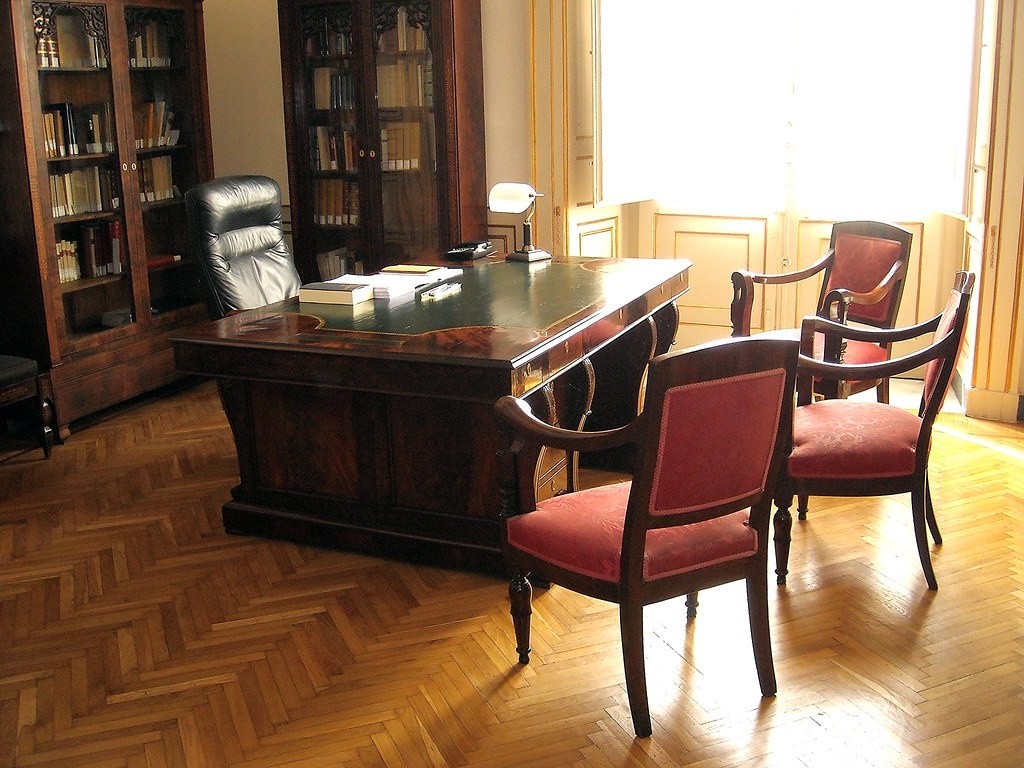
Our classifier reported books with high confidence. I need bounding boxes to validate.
[298,265,463,306]
[32,13,183,281]
[307,8,426,171]
[313,179,360,224]
[317,243,366,281]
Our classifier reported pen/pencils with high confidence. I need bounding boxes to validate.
[420,282,462,298]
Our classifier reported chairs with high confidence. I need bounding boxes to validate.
[770,268,974,590]
[729,219,928,408]
[493,336,805,741]
[178,173,316,317]
[161,244,694,591]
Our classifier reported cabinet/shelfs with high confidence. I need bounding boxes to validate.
[275,0,488,286]
[0,0,223,444]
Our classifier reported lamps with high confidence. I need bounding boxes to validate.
[485,179,553,264]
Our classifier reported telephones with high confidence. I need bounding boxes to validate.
[445,240,493,261]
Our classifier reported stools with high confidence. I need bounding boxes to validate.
[0,352,59,463]
